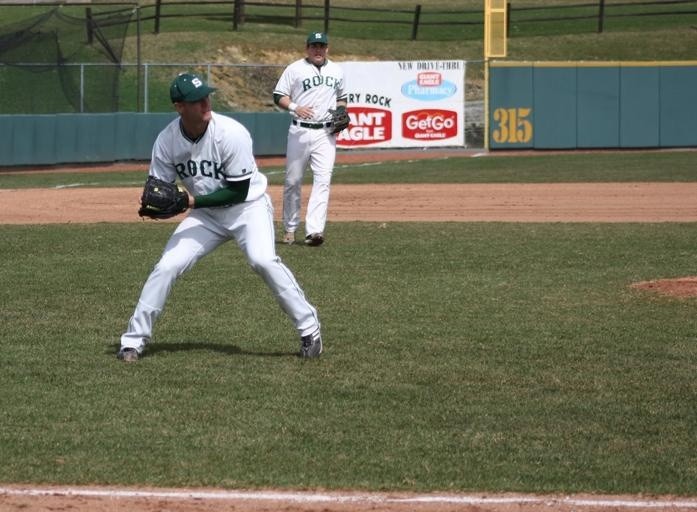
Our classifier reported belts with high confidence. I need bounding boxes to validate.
[293,120,333,128]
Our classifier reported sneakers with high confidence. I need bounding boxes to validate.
[283,230,296,245]
[305,232,324,245]
[301,323,323,358]
[118,347,139,362]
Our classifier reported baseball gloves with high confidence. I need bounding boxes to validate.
[139,175,188,220]
[328,109,350,135]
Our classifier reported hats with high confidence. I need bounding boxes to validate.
[170,73,218,101]
[307,31,329,45]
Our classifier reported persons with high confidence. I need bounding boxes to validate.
[114,71,323,363]
[273,30,351,245]
